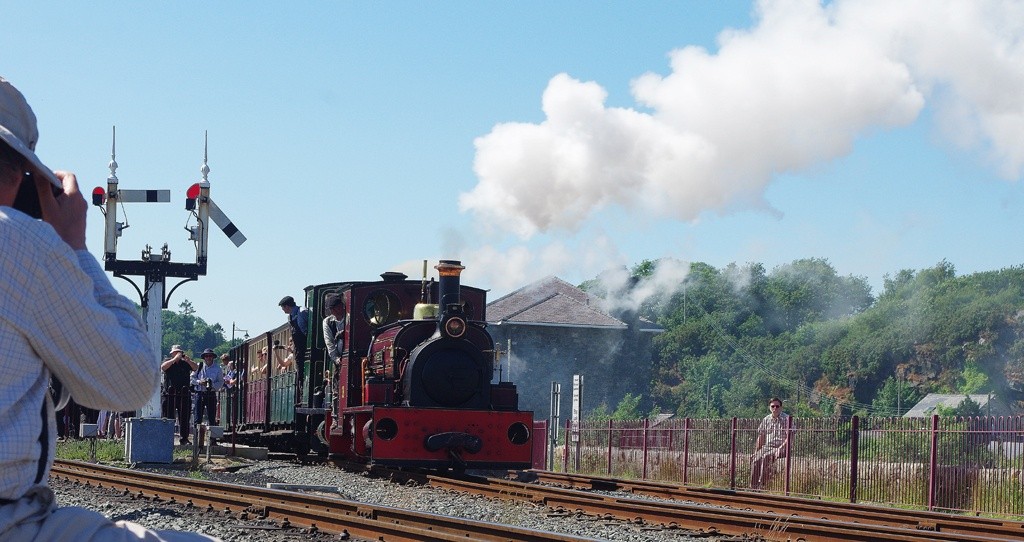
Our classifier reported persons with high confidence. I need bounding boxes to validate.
[279,296,309,408]
[370,306,386,326]
[323,297,346,430]
[988,471,1021,487]
[751,398,794,489]
[252,337,298,379]
[161,344,245,445]
[0,78,224,542]
[48,377,127,440]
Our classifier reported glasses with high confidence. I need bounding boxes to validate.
[262,353,268,356]
[205,355,213,358]
[771,405,779,408]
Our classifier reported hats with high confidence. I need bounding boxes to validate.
[170,345,183,354]
[279,295,294,306]
[200,348,217,359]
[325,293,341,309]
[0,75,64,193]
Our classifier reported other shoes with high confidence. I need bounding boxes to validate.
[180,439,192,446]
[330,419,338,430]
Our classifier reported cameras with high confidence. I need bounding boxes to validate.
[14,174,64,219]
[278,345,286,349]
[206,378,212,389]
[182,355,185,357]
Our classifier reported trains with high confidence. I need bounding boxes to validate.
[190,259,533,470]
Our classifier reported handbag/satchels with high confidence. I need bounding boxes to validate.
[190,385,197,393]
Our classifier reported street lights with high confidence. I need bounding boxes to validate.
[232,322,249,347]
